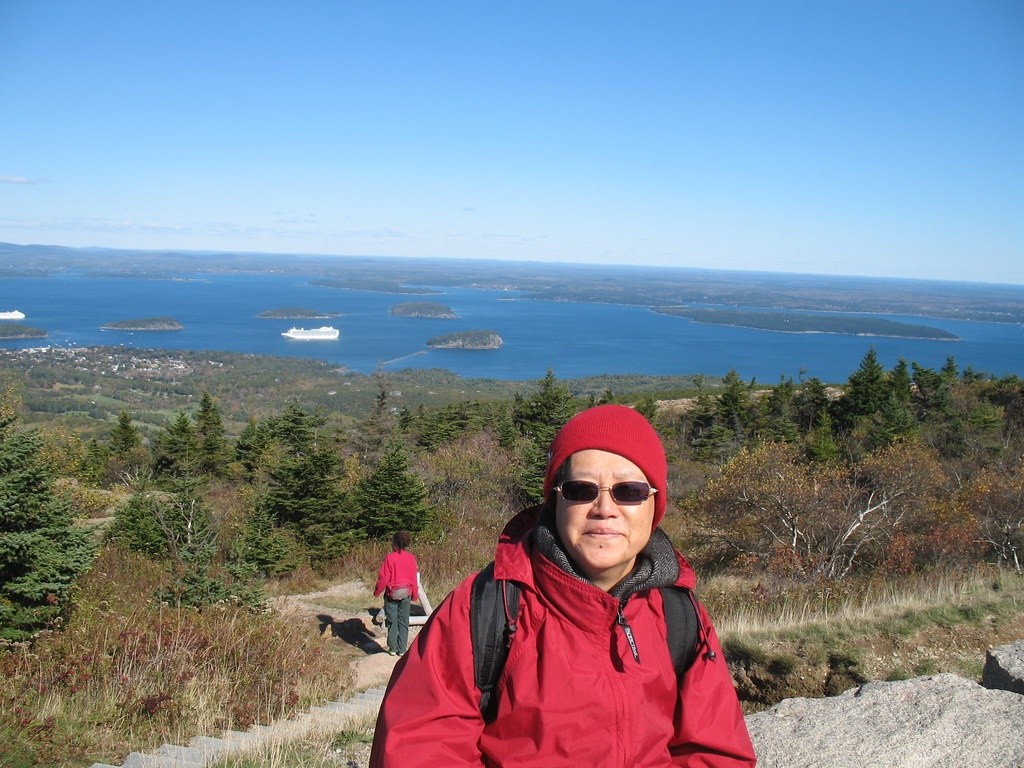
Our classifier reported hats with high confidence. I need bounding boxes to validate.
[543,404,666,533]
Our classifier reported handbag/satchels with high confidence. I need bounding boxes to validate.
[391,587,408,600]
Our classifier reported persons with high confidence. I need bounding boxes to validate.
[373,404,760,768]
[373,532,419,656]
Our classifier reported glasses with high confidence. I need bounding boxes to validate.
[552,479,659,503]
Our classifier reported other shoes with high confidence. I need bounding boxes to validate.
[399,653,404,656]
[388,648,396,656]
